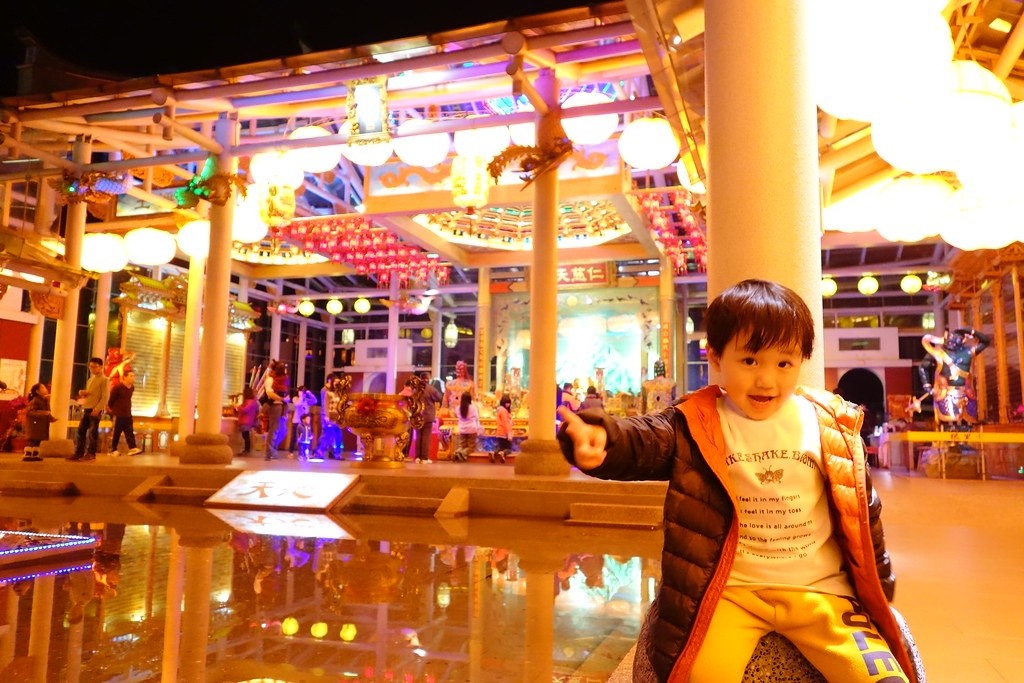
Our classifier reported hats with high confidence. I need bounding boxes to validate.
[420,371,430,379]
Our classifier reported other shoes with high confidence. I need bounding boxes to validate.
[489,451,495,463]
[66,451,84,461]
[421,458,432,464]
[459,451,467,460]
[79,452,96,461]
[456,454,463,462]
[108,450,121,456]
[128,447,143,456]
[415,458,421,464]
[238,450,345,462]
[498,450,506,463]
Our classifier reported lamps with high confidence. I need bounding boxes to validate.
[231,93,705,243]
[437,580,450,609]
[299,298,371,316]
[282,615,359,642]
[80,219,210,273]
[812,0,1024,299]
[443,319,458,348]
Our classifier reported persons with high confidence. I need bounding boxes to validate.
[238,388,259,456]
[68,358,110,462]
[443,360,476,406]
[107,370,141,459]
[259,360,316,461]
[22,383,58,462]
[102,346,136,379]
[488,396,512,464]
[918,327,991,451]
[398,372,443,466]
[311,375,345,460]
[450,391,480,464]
[556,382,604,441]
[556,278,917,683]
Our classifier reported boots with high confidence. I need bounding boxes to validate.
[32,446,43,461]
[23,446,33,460]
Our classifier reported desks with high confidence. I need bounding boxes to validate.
[438,417,527,461]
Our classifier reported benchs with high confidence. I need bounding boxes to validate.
[67,416,242,455]
